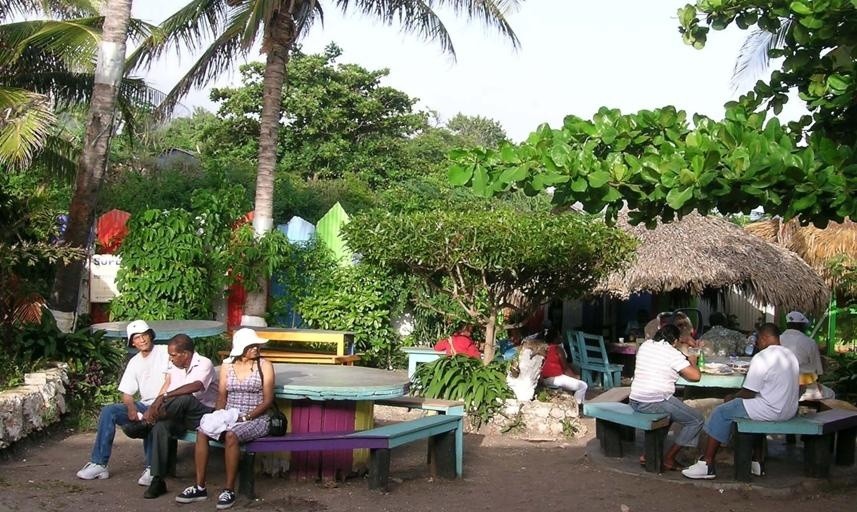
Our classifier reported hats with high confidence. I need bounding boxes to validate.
[127,319,156,349]
[223,328,269,364]
[786,311,810,323]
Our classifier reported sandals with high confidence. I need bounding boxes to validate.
[639,455,647,467]
[664,461,685,471]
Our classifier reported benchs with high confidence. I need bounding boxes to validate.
[582,387,671,473]
[732,398,856,480]
[218,350,360,369]
[165,415,464,499]
[373,395,465,416]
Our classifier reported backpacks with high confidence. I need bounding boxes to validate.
[254,355,287,437]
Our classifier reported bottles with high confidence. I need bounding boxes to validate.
[729,341,738,365]
[744,331,757,355]
[696,347,708,372]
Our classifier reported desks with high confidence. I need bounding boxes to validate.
[213,363,410,481]
[231,328,354,356]
[400,346,484,378]
[90,319,224,359]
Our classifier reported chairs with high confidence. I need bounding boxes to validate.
[578,330,624,391]
[566,329,601,388]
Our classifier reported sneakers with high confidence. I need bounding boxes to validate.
[76,462,109,480]
[144,477,167,498]
[751,457,766,476]
[175,485,207,503]
[123,420,149,440]
[681,461,716,479]
[138,468,153,485]
[216,488,236,509]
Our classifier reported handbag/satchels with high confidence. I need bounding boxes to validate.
[257,357,288,437]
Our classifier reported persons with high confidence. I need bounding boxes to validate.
[75,321,172,487]
[681,323,800,479]
[674,314,698,355]
[123,332,219,499]
[695,312,750,357]
[539,330,588,410]
[433,322,481,365]
[779,311,835,400]
[627,324,706,472]
[173,326,275,509]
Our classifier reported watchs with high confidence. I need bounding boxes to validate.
[163,392,168,403]
